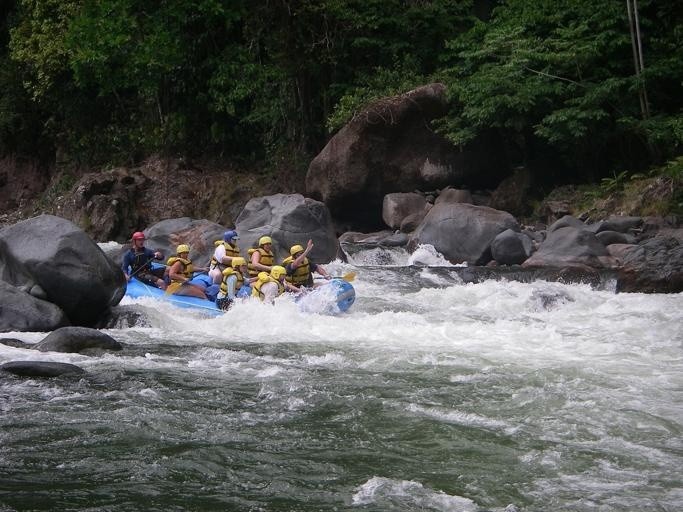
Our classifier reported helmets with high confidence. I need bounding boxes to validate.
[269,266,288,280]
[290,243,304,256]
[258,235,273,247]
[131,230,146,240]
[176,243,190,255]
[231,255,248,270]
[224,230,239,243]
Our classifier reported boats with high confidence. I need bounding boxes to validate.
[122,259,356,320]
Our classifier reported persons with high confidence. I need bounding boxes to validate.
[283,239,331,288]
[215,256,258,311]
[246,236,274,287]
[208,230,241,284]
[164,244,209,299]
[251,265,300,303]
[121,231,171,290]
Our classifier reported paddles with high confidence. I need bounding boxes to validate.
[314,273,356,281]
[166,270,207,296]
